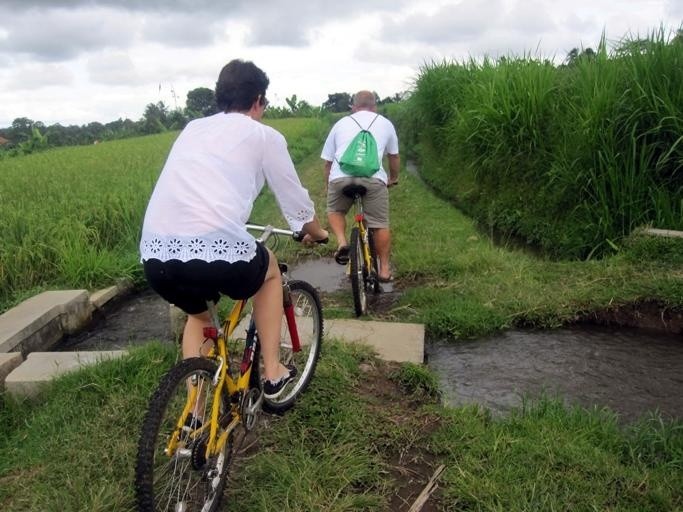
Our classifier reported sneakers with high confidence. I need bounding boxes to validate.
[263,364,298,399]
[183,412,202,433]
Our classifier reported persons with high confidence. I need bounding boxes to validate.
[140,60,325,431]
[321,90,401,283]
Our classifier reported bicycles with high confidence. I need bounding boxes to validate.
[136,221,328,512]
[339,183,398,316]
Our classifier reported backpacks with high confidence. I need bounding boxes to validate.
[334,114,380,178]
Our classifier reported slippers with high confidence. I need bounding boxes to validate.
[335,246,350,265]
[378,274,394,282]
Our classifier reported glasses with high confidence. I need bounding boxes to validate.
[262,97,269,109]
